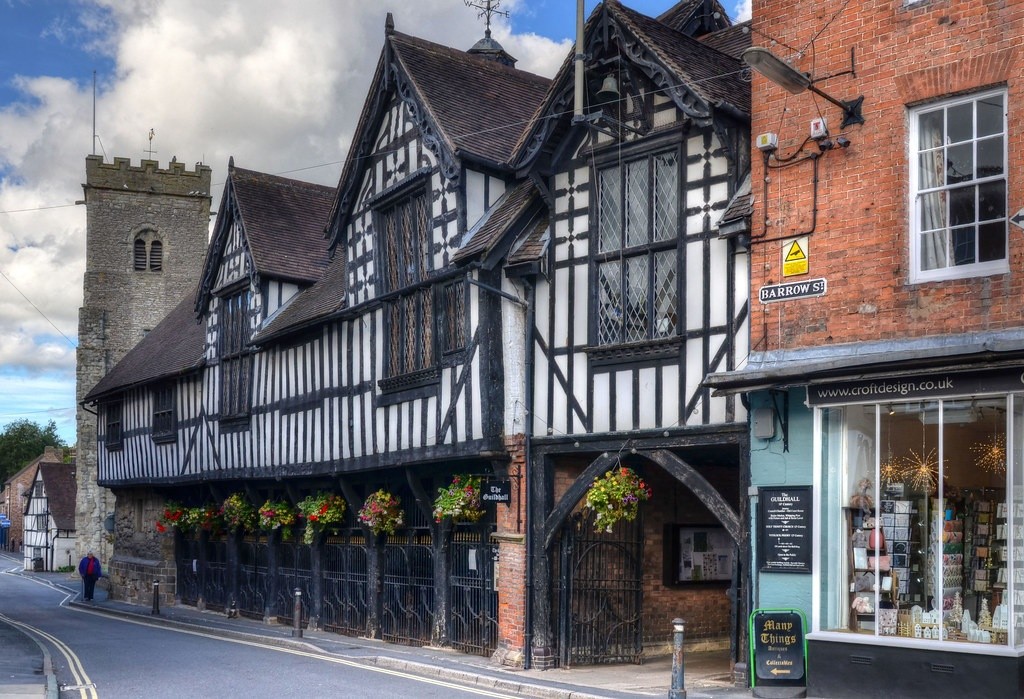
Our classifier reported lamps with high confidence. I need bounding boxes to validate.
[970,406,1019,475]
[740,45,865,131]
[867,415,911,490]
[900,411,948,493]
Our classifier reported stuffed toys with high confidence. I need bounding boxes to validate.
[850,517,876,613]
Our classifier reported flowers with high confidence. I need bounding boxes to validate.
[212,494,256,532]
[586,466,654,535]
[187,502,222,539]
[256,500,295,541]
[297,490,348,546]
[356,490,407,535]
[152,499,188,535]
[432,473,487,526]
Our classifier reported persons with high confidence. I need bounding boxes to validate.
[78,551,103,601]
[10,537,22,552]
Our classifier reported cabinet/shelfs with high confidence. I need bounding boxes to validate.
[879,485,1024,641]
[842,506,899,634]
